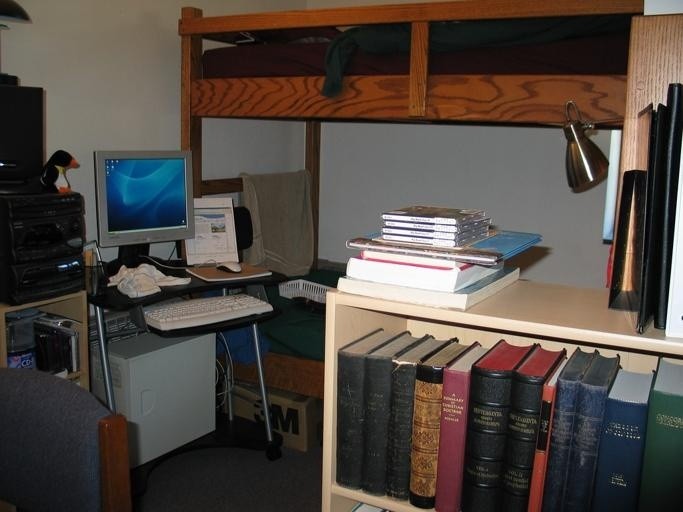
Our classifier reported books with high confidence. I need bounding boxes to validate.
[335,328,682,511]
[336,205,542,312]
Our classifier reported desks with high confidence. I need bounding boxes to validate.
[88,260,288,461]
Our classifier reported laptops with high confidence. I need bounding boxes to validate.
[185,197,272,283]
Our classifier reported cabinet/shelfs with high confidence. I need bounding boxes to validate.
[322,280,683,512]
[0,290,90,391]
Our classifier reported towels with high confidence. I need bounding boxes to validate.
[239,169,315,277]
[107,263,191,298]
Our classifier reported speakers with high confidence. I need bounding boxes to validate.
[234,206,253,251]
[0,84,43,196]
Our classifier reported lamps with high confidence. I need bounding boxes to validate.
[564,100,624,188]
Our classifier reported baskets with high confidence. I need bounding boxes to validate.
[279,279,332,304]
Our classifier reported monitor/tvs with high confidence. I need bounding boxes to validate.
[94,150,195,276]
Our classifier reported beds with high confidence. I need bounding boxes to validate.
[179,0,643,400]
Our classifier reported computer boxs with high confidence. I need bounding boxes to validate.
[90,330,216,470]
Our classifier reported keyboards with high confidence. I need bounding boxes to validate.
[143,293,273,332]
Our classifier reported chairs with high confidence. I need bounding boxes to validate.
[0,368,133,512]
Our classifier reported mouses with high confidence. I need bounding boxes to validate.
[216,260,242,273]
